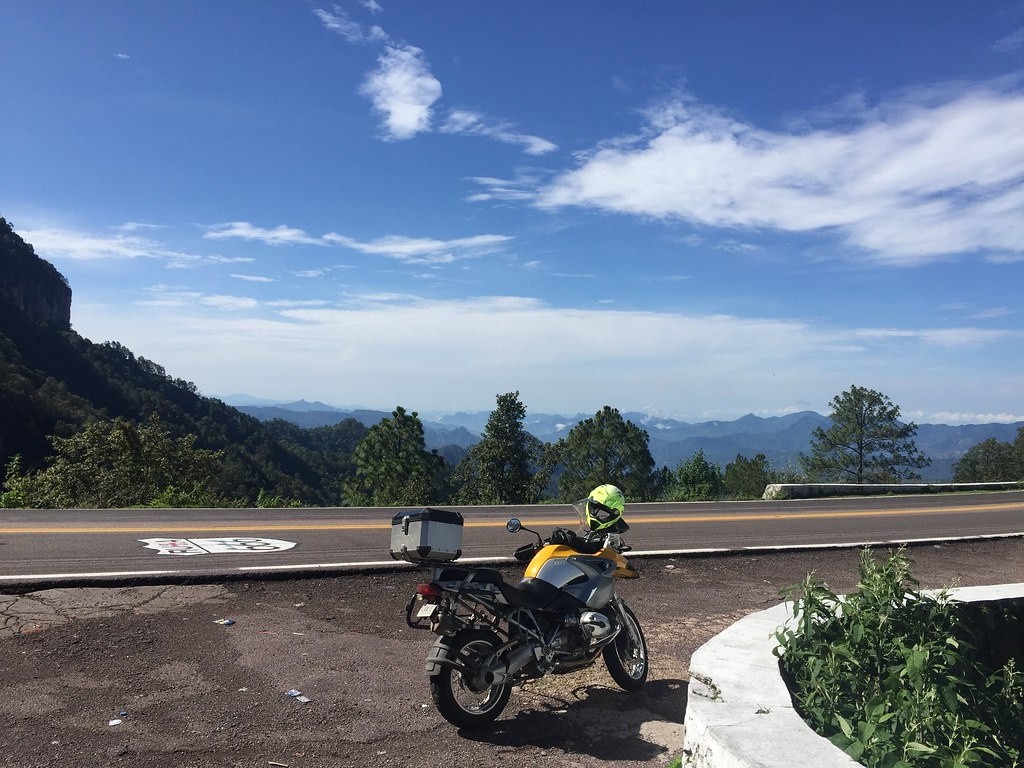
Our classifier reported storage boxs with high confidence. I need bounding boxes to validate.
[391,508,464,563]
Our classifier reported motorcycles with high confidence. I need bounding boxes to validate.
[389,498,650,730]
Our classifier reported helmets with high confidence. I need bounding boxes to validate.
[585,484,625,531]
